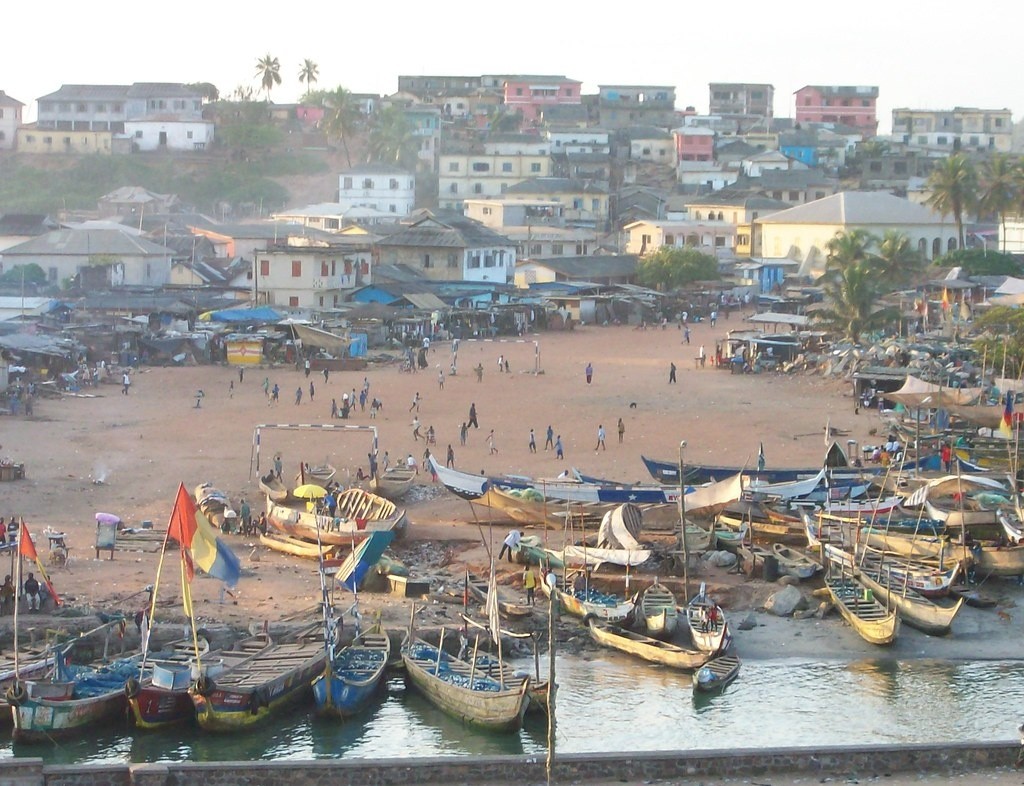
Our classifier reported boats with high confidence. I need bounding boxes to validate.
[333,529,398,591]
[258,529,336,560]
[310,528,391,719]
[375,464,419,501]
[456,572,560,714]
[186,513,345,737]
[264,491,408,546]
[426,451,717,530]
[258,472,288,502]
[306,461,337,489]
[466,569,534,621]
[643,407,1024,648]
[5,625,211,746]
[587,616,719,671]
[195,482,234,529]
[691,652,742,695]
[540,515,637,627]
[125,619,275,731]
[399,599,533,733]
[686,582,735,655]
[514,539,653,574]
[0,626,76,723]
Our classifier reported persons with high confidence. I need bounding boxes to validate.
[523,565,537,605]
[586,362,593,384]
[723,292,750,307]
[10,380,34,415]
[240,499,266,537]
[660,311,691,344]
[700,345,705,364]
[710,311,717,327]
[871,440,900,463]
[324,481,344,517]
[121,372,131,395]
[572,571,586,595]
[701,603,717,632]
[957,432,968,447]
[595,425,606,452]
[498,529,524,563]
[0,573,53,616]
[617,418,626,444]
[229,336,562,483]
[942,441,951,470]
[669,363,677,383]
[0,515,18,543]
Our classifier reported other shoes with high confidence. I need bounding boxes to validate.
[35,609,39,613]
[29,609,32,614]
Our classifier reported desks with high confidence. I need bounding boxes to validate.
[847,440,858,459]
[863,451,872,461]
[695,357,704,369]
[48,534,66,549]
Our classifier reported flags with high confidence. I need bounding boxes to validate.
[999,392,1014,440]
[21,522,61,605]
[171,485,239,587]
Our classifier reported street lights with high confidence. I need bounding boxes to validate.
[252,247,260,308]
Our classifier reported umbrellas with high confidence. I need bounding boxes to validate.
[293,484,327,498]
